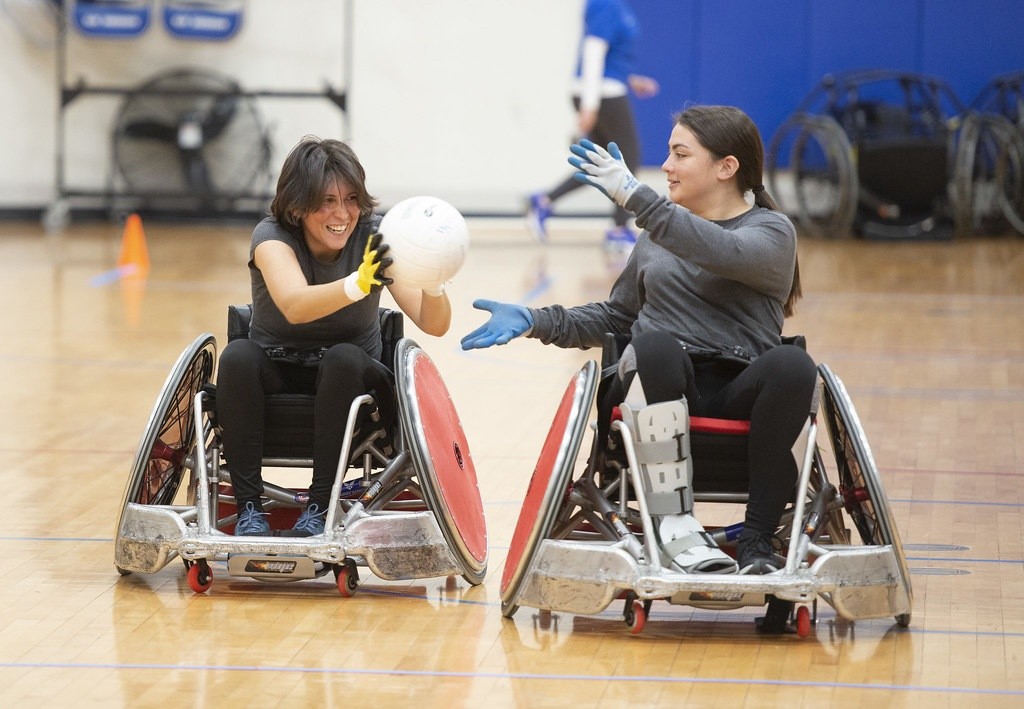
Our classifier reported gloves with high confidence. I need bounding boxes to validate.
[461,299,533,351]
[343,233,392,303]
[423,283,445,297]
[568,138,643,208]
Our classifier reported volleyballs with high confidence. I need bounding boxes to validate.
[378,195,468,291]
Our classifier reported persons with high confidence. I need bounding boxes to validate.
[461,104,816,575]
[528,0,660,251]
[216,137,450,536]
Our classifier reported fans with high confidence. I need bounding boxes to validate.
[107,67,276,224]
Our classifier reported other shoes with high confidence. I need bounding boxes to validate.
[734,532,787,575]
[606,226,637,246]
[293,503,328,535]
[234,501,271,536]
[528,192,552,242]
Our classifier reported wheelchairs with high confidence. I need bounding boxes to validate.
[112,301,491,600]
[499,328,916,641]
[771,63,1024,247]
